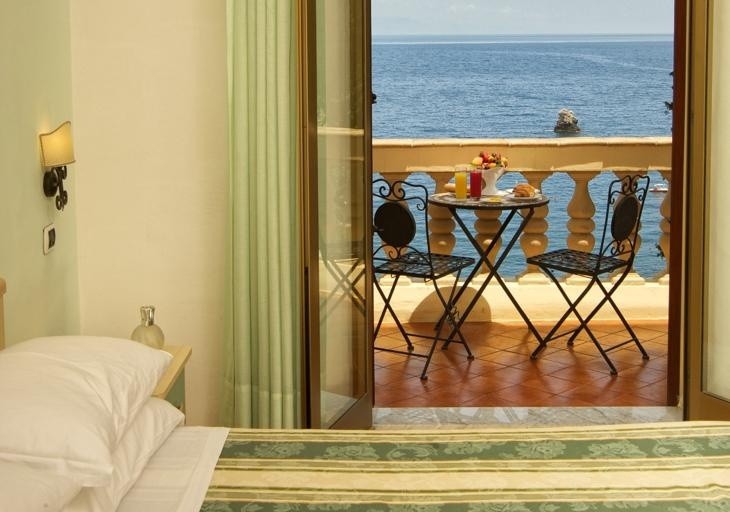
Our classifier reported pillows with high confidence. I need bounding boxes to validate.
[1,333,174,483]
[63,397,184,512]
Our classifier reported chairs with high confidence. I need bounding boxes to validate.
[374,179,474,380]
[526,174,648,374]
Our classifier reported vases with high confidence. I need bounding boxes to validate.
[481,169,505,196]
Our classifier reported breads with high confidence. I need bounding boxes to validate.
[514,183,536,197]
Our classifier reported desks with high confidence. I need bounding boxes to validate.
[427,191,548,349]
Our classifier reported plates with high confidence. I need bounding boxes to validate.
[506,189,541,194]
[509,193,541,201]
[478,194,508,205]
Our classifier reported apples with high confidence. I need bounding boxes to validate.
[471,151,508,169]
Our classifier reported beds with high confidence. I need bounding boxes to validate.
[0,278,730,511]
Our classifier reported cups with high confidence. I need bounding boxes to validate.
[470,166,483,200]
[454,166,468,201]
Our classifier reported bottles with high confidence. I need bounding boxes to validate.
[131,305,164,350]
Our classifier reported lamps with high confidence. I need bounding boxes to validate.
[38,119,76,212]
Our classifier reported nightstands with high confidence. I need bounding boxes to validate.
[148,344,194,426]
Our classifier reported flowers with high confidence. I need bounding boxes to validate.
[468,151,510,168]
